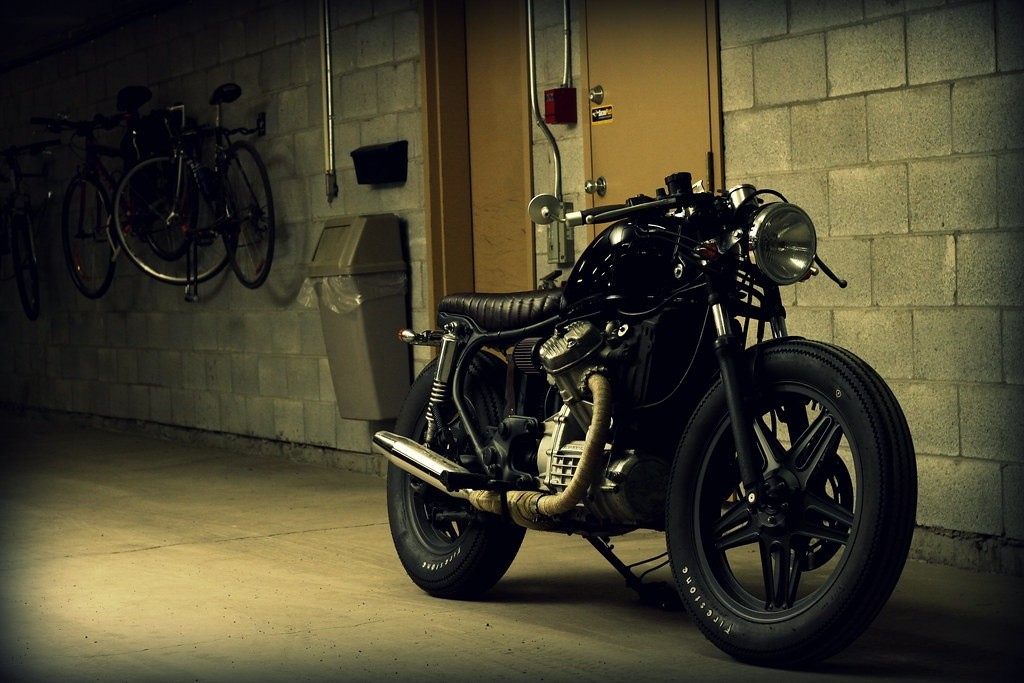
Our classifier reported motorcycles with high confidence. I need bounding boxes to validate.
[372,172,917,668]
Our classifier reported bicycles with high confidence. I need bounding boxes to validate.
[110,84,275,304]
[0,138,61,321]
[31,85,199,300]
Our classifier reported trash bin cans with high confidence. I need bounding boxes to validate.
[305,213,412,423]
